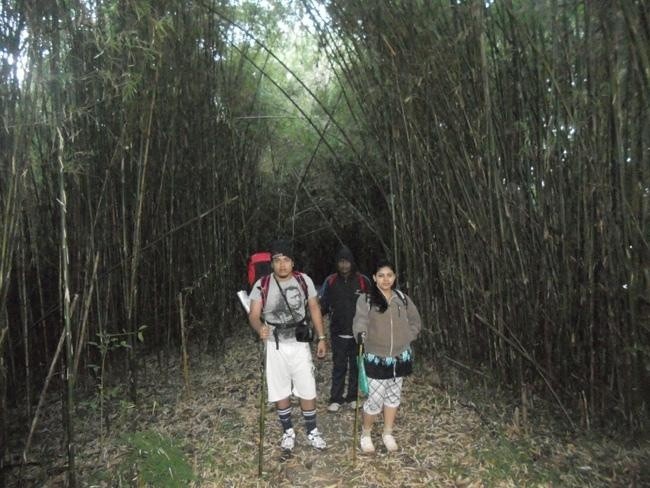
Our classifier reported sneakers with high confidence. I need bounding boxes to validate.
[280,427,296,453]
[381,433,399,452]
[327,402,342,413]
[305,426,328,451]
[360,433,375,454]
[345,401,363,411]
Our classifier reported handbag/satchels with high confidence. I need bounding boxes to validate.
[296,324,314,342]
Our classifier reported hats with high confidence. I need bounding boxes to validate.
[270,238,295,261]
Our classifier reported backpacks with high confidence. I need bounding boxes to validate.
[247,252,308,309]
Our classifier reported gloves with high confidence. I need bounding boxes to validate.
[358,331,366,345]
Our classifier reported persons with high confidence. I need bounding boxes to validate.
[247,239,328,454]
[351,261,422,454]
[307,245,372,412]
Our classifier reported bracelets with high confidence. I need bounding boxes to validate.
[318,336,327,340]
